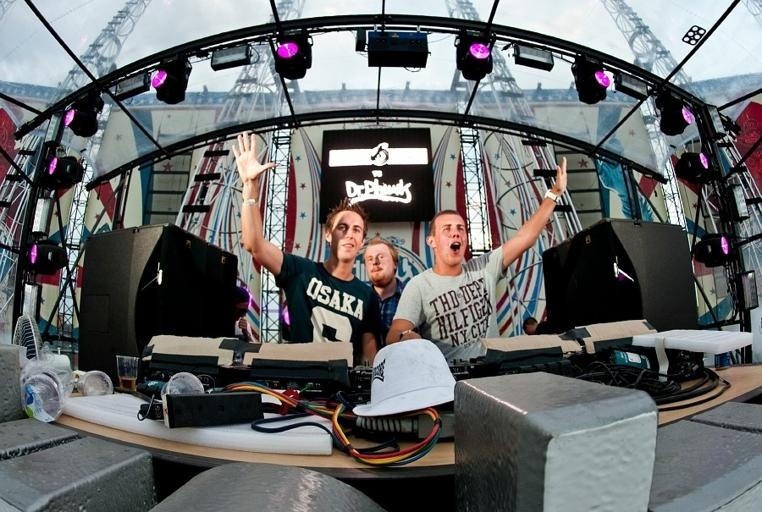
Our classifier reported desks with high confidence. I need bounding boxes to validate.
[50,363,762,485]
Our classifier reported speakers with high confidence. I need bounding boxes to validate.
[542,219,697,331]
[79,224,237,387]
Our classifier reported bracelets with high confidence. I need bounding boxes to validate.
[242,197,260,206]
[399,329,413,340]
[247,334,251,337]
[544,189,559,203]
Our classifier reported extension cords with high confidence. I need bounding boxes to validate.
[162,391,263,428]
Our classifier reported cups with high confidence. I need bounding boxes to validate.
[115,355,139,389]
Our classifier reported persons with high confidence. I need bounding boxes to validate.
[232,133,390,365]
[234,286,259,342]
[386,157,568,367]
[522,317,554,334]
[364,234,404,349]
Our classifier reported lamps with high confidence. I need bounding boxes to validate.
[360,26,433,72]
[655,91,730,269]
[114,70,150,107]
[568,52,610,106]
[271,28,318,81]
[510,39,558,74]
[25,92,104,275]
[613,67,650,105]
[450,30,498,85]
[209,40,260,72]
[150,54,194,108]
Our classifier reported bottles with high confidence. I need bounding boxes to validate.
[20,366,113,423]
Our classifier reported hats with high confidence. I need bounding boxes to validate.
[353,339,456,416]
